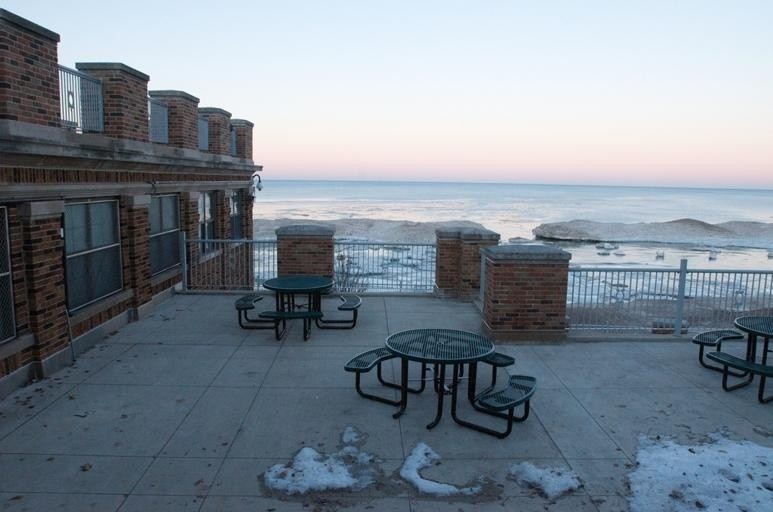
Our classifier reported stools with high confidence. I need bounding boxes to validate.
[450,361,538,440]
[235,292,275,330]
[342,346,425,407]
[317,291,361,331]
[693,330,749,379]
[707,351,772,403]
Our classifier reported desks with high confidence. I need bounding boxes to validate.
[719,316,773,403]
[264,273,335,343]
[385,327,496,431]
